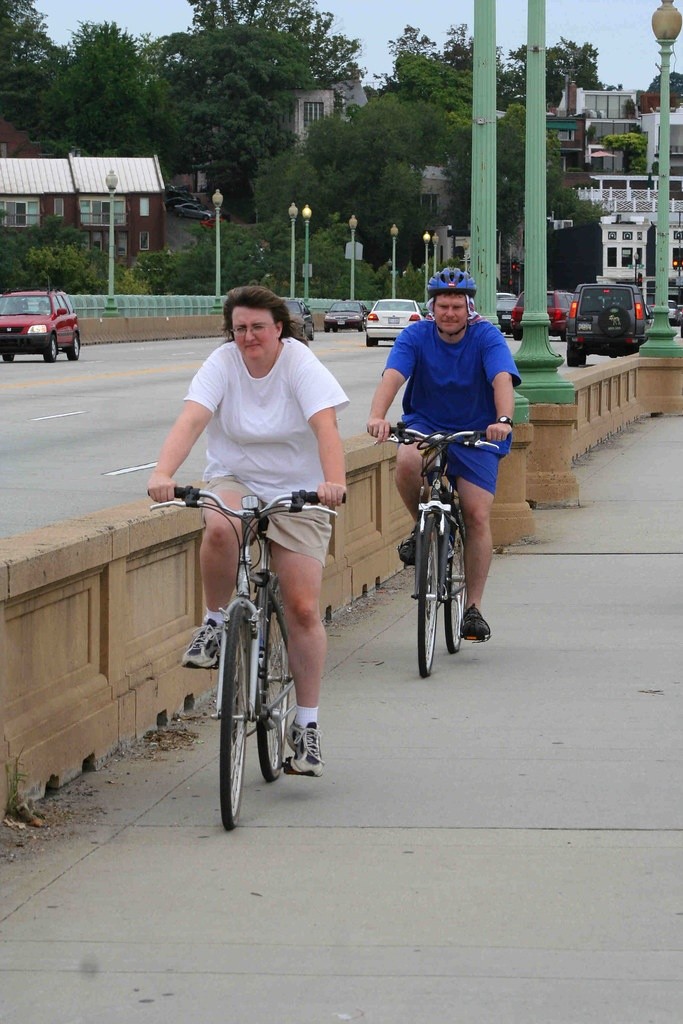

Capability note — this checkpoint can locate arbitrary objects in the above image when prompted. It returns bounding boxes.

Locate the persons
[146,286,350,778]
[39,301,47,310]
[366,269,523,640]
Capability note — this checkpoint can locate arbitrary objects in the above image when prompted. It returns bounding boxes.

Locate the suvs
[495,284,683,368]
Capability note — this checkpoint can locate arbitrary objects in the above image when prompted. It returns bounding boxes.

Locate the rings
[498,438,501,439]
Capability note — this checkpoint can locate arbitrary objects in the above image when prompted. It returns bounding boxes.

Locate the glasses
[230,323,274,336]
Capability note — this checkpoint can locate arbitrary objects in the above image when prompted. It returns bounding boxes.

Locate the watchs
[496,416,513,427]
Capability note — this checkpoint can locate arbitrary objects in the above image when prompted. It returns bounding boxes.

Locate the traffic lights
[673,248,683,267]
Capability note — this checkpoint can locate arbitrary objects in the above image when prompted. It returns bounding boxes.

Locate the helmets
[427,268,477,297]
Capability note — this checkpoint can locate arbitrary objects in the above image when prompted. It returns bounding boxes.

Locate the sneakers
[399,525,416,565]
[460,603,490,638]
[181,615,222,668]
[287,714,325,776]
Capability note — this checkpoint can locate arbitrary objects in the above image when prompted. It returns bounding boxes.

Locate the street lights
[301,205,313,313]
[634,252,638,285]
[639,0,683,359]
[288,203,298,299]
[464,241,469,273]
[423,232,430,302]
[350,215,357,301]
[390,224,398,299]
[211,189,225,314]
[101,168,122,317]
[432,233,439,277]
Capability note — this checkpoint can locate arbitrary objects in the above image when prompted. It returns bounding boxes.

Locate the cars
[366,299,425,347]
[0,289,81,362]
[282,298,314,341]
[323,302,371,332]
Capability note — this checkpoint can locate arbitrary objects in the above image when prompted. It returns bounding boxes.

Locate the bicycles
[367,422,499,678]
[147,485,347,831]
